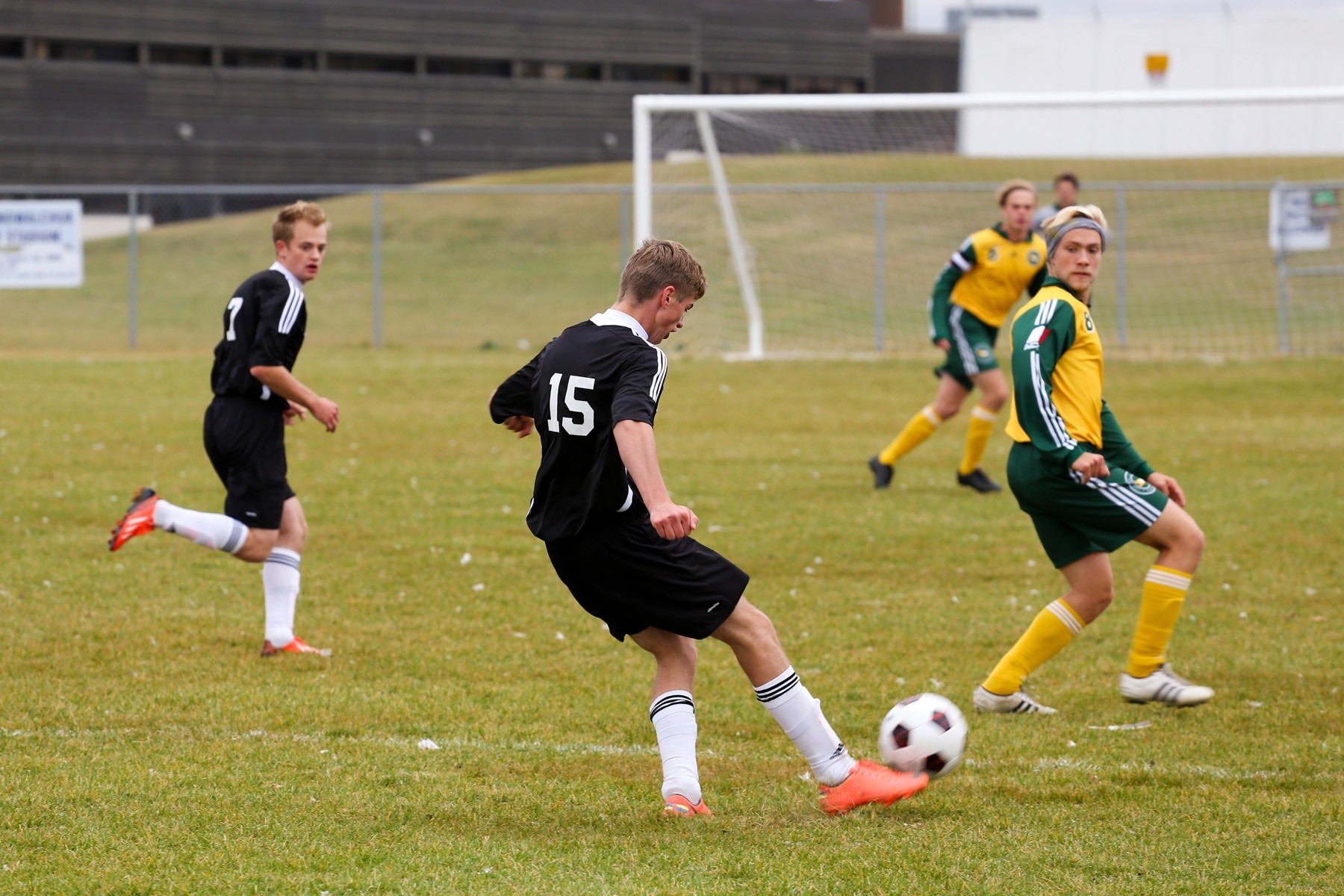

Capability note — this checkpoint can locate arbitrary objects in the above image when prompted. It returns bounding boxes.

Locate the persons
[865,179,1049,494]
[488,235,929,818]
[106,201,344,661]
[1033,171,1080,229]
[970,206,1215,719]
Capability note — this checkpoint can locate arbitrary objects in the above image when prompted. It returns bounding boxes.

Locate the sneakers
[260,635,334,659]
[972,685,1058,715]
[818,760,930,816]
[108,486,161,552]
[1120,662,1216,708]
[867,454,895,489]
[662,793,714,818]
[956,466,1004,492]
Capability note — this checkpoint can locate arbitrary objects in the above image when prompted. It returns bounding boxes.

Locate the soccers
[879,694,967,780]
